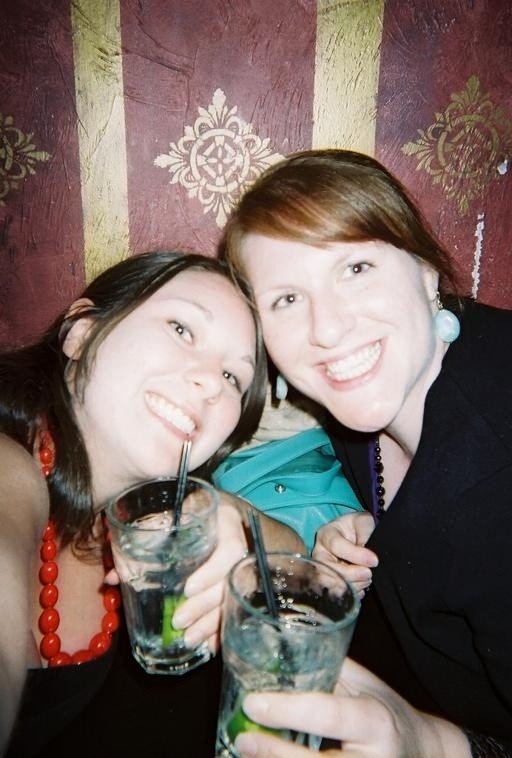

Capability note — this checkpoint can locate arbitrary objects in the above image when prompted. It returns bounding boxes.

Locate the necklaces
[372,432,386,520]
[32,427,121,671]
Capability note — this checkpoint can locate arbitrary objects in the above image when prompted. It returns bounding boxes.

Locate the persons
[1,238,310,758]
[214,147,512,756]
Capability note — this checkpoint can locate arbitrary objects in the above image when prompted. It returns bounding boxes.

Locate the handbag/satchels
[208,427,367,552]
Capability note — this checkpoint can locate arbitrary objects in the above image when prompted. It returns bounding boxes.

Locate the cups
[104,474,223,676]
[213,550,361,756]
